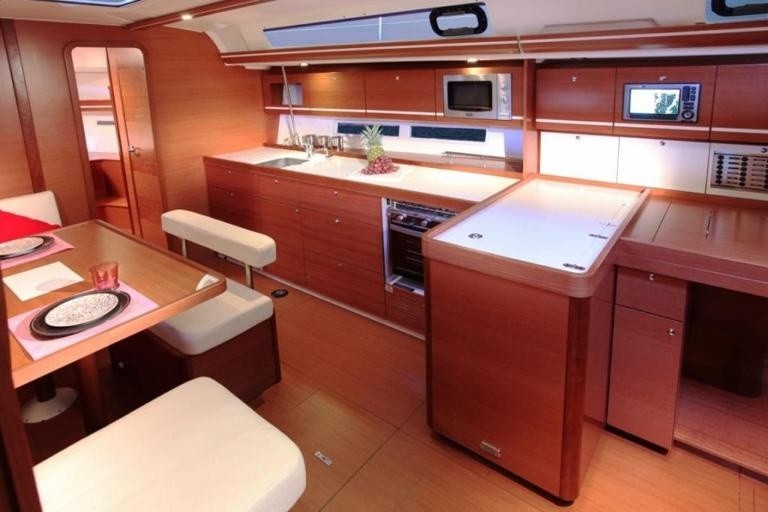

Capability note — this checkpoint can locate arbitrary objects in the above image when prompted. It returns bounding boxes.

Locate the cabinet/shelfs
[302,173,384,324]
[261,62,525,132]
[605,264,688,452]
[203,153,306,294]
[533,62,768,144]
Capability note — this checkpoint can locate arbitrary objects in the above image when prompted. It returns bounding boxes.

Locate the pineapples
[359,122,385,162]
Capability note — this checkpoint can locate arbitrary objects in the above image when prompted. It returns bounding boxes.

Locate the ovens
[380,196,462,296]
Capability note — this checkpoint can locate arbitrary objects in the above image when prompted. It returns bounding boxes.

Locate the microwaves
[442,74,513,123]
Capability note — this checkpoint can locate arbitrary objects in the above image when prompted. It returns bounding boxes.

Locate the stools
[32,376,306,512]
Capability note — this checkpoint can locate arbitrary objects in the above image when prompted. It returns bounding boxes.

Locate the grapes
[361,154,399,176]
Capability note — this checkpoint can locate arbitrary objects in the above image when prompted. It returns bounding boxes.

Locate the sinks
[256,157,308,169]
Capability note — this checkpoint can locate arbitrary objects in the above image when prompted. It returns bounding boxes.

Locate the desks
[0,219,226,429]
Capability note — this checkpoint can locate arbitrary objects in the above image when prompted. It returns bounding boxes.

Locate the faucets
[301,142,314,157]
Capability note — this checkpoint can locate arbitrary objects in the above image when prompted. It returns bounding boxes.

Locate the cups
[304,134,344,152]
[87,260,120,292]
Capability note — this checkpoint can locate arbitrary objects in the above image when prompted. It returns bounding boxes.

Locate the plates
[43,292,117,328]
[0,236,44,257]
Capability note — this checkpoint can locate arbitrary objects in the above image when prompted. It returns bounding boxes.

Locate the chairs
[0,189,62,245]
[108,208,281,403]
